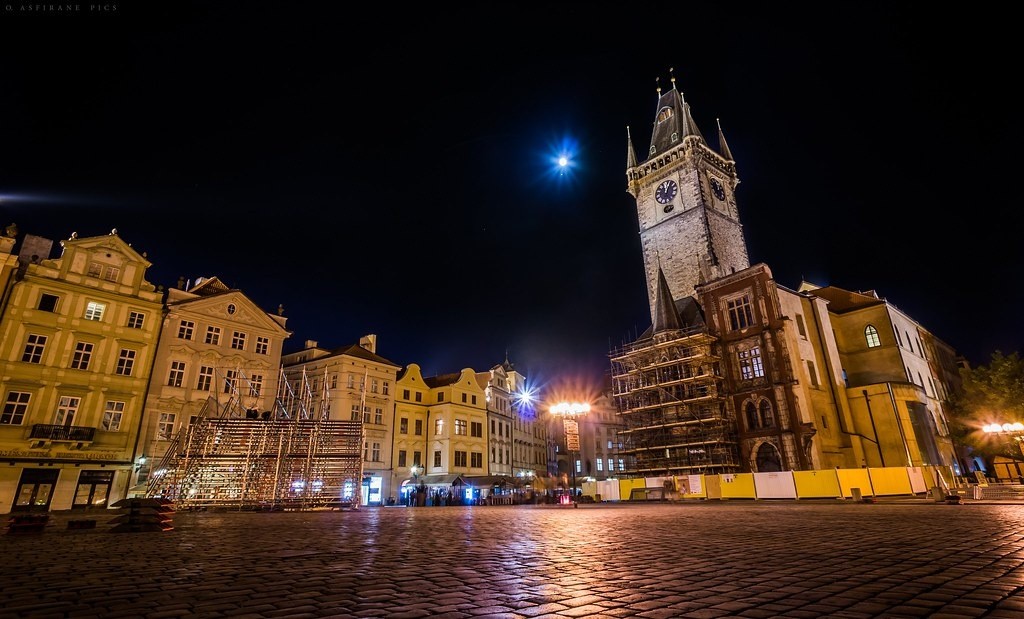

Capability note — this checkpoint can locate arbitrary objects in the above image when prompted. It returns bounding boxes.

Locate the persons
[410,489,582,506]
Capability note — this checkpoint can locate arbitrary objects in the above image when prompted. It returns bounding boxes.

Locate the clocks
[655,180,678,204]
[710,178,725,202]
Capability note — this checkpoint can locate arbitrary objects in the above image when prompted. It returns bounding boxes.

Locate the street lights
[547,400,592,508]
[510,393,530,478]
[982,422,1024,485]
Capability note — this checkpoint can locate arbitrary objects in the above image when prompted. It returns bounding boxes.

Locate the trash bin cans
[931,486,944,501]
[595,494,600,502]
[850,488,862,501]
